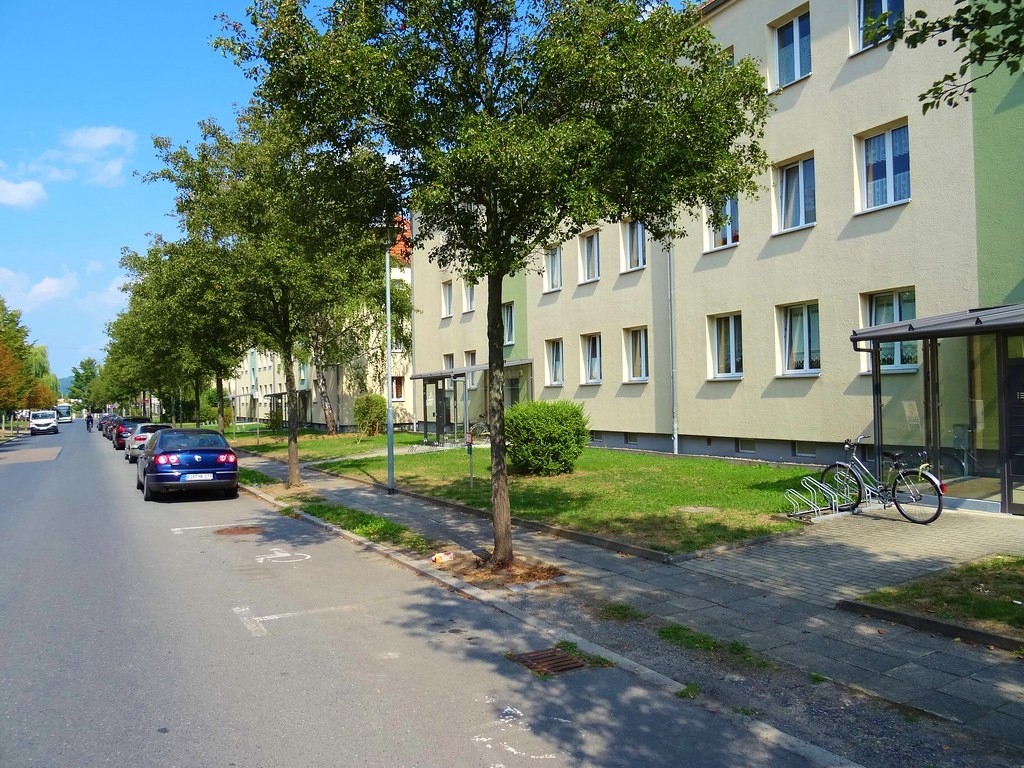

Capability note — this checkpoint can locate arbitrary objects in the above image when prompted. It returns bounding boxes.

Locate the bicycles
[941,430,1000,480]
[85,420,93,432]
[820,435,946,524]
[469,414,490,445]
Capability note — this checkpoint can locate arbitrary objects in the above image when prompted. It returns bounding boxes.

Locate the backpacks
[87,414,91,417]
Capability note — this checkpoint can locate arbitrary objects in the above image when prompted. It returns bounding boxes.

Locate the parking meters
[466,432,473,490]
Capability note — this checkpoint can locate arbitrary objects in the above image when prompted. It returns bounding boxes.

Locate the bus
[55,404,73,423]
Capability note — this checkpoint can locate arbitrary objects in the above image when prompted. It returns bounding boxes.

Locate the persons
[85,411,93,430]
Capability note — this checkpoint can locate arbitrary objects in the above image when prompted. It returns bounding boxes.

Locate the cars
[137,429,239,501]
[97,413,152,450]
[125,423,175,463]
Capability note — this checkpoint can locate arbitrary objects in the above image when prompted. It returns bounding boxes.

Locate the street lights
[376,224,407,495]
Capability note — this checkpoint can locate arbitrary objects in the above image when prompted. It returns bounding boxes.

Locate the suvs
[29,411,58,436]
